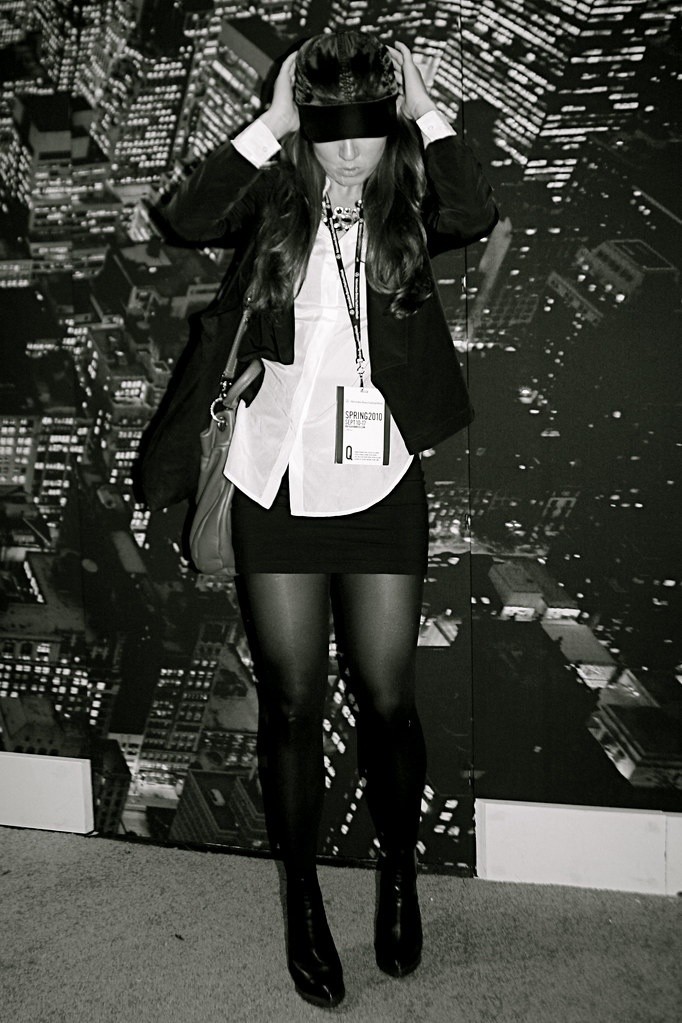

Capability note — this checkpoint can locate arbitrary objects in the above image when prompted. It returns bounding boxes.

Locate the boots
[277,859,346,1007]
[374,847,424,977]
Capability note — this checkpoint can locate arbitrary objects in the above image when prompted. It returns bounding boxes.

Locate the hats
[294,28,399,142]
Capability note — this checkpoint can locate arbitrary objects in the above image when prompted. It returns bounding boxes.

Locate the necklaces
[321,202,363,230]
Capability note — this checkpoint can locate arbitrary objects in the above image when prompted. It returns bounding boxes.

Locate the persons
[159,31,499,1006]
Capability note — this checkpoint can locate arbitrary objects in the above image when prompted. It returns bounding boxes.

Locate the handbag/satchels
[128,193,269,511]
[188,401,237,576]
